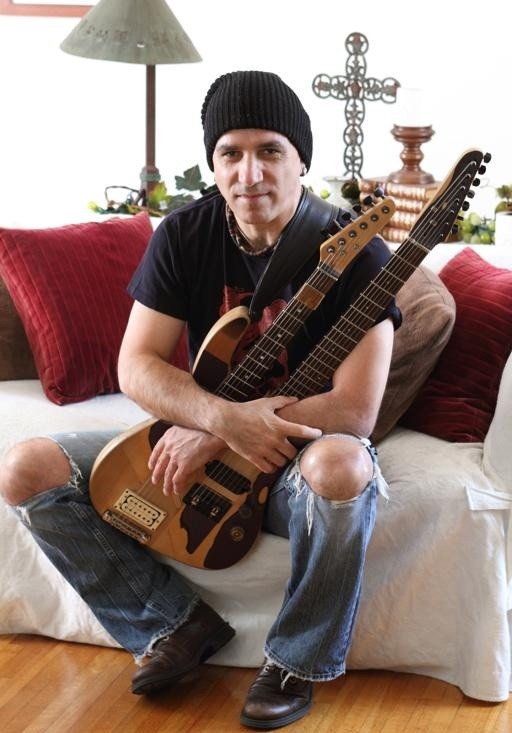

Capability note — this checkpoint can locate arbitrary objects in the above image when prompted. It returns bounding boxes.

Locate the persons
[1,70,404,730]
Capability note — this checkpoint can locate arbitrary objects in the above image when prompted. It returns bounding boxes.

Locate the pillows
[0,210,191,406]
[368,246,512,445]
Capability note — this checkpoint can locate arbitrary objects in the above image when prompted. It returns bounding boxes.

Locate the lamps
[59,0,204,209]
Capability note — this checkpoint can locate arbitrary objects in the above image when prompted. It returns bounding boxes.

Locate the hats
[201,71,312,169]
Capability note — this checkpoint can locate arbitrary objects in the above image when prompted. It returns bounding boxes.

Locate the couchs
[0,242,512,704]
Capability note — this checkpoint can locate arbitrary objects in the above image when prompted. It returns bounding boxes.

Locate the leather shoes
[130,598,237,694]
[240,658,311,729]
